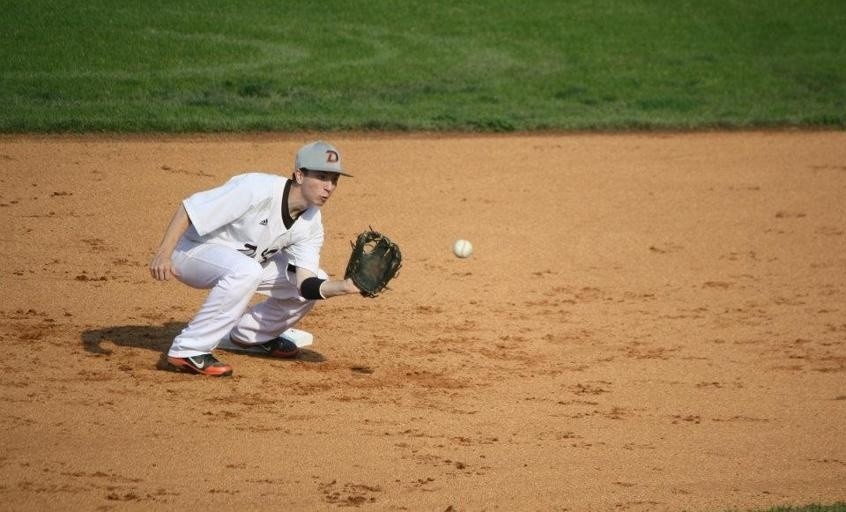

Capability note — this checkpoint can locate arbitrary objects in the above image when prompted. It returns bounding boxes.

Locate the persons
[149,138,363,379]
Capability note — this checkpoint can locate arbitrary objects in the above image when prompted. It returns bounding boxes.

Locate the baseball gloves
[344,230,401,297]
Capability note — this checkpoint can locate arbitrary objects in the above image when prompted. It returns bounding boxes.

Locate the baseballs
[454,239,472,257]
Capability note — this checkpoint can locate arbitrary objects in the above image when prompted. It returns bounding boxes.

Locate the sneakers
[167,349,233,378]
[227,326,298,359]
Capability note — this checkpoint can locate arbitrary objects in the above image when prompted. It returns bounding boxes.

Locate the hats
[292,139,355,180]
